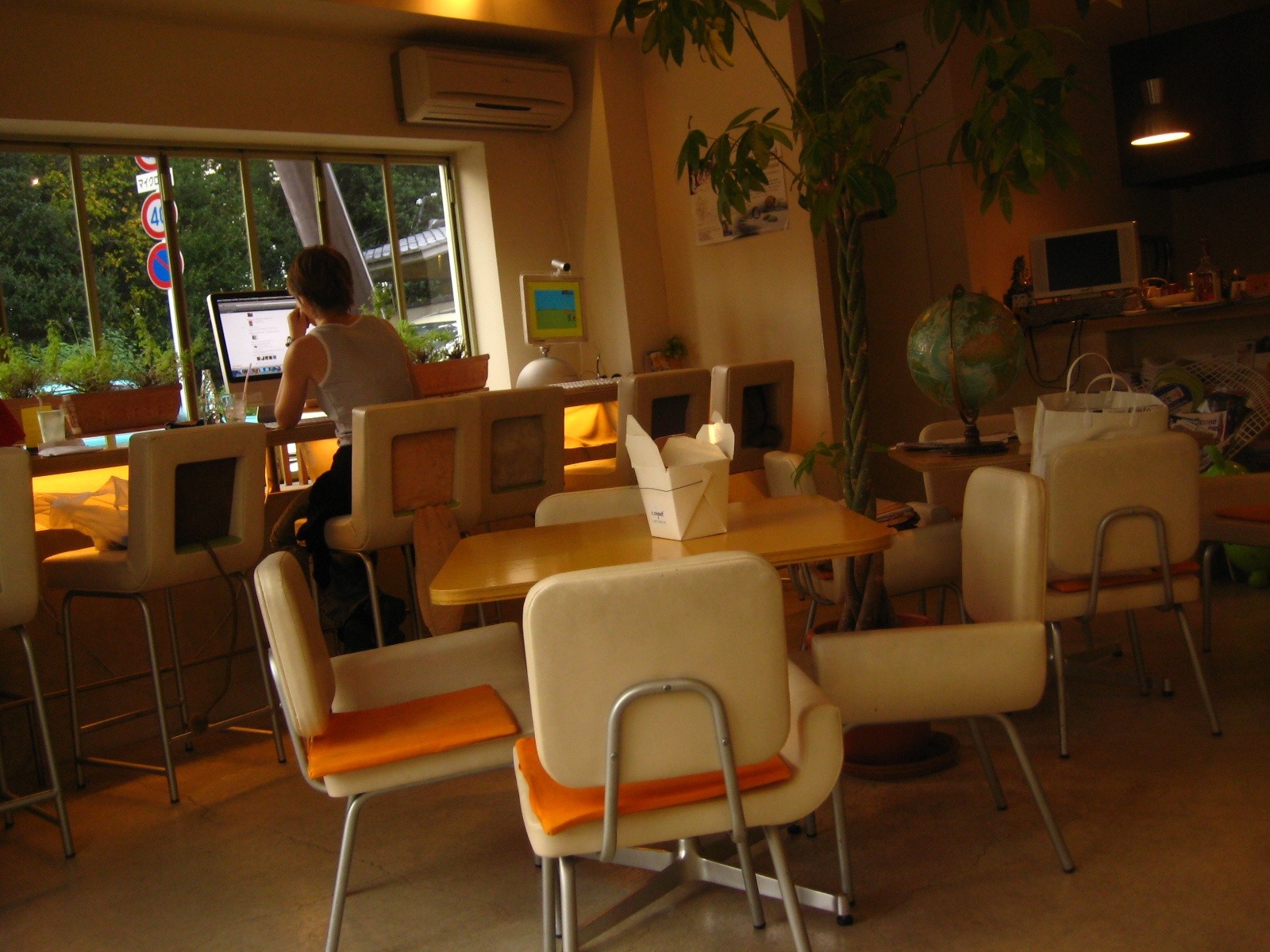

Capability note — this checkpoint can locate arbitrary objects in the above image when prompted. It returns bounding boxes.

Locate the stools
[309,394,497,656]
[712,352,799,474]
[476,380,571,534]
[41,418,282,805]
[0,446,79,866]
[566,362,719,490]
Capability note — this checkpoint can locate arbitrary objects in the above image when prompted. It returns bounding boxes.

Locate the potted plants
[0,298,184,441]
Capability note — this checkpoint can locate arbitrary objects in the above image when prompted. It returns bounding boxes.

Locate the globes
[906,283,1027,456]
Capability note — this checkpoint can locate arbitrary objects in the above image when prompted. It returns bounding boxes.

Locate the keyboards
[548,375,621,393]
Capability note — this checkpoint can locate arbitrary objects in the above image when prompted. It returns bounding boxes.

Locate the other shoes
[333,594,407,656]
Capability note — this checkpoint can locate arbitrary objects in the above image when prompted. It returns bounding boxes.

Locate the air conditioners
[389,44,576,133]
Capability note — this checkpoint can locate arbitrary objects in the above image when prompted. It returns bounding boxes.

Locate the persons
[271,244,412,655]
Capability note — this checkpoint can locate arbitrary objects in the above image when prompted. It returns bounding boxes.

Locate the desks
[884,433,1123,698]
[423,496,898,952]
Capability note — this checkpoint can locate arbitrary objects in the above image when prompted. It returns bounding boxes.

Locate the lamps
[1125,0,1198,150]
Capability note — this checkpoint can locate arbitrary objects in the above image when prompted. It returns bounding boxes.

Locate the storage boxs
[609,400,738,540]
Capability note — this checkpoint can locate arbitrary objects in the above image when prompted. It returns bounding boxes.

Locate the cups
[222,392,247,423]
[36,409,65,443]
[1012,405,1036,444]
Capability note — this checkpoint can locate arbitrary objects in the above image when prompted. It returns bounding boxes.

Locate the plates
[1147,292,1195,309]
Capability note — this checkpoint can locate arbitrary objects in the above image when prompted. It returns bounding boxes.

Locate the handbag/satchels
[1028,353,1169,583]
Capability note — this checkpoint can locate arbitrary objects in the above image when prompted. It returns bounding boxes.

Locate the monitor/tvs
[520,270,591,344]
[206,290,317,384]
[1027,220,1143,298]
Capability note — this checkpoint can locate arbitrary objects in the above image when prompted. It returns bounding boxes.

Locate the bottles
[200,368,221,425]
[1193,238,1221,301]
[59,395,83,438]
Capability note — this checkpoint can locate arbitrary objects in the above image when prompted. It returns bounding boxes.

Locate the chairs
[239,542,558,952]
[506,542,845,952]
[1029,431,1224,761]
[756,442,968,651]
[1195,463,1270,658]
[803,467,1084,901]
[532,482,646,529]
